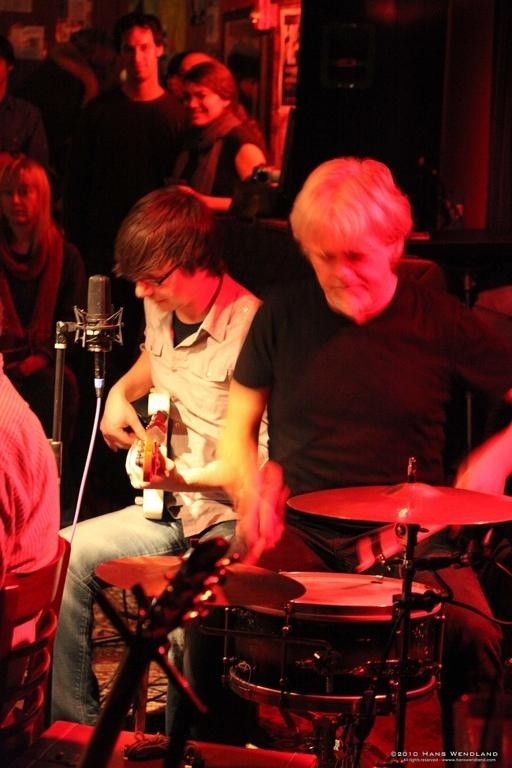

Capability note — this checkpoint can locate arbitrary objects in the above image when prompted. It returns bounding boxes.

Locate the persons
[1,353,60,725]
[48,184,270,741]
[0,13,284,522]
[154,153,512,740]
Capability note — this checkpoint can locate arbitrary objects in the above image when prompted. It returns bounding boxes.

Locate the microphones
[84,273,114,393]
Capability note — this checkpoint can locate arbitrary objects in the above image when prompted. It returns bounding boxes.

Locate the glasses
[149,261,183,289]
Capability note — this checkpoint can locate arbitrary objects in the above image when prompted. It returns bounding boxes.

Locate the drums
[227,572,442,700]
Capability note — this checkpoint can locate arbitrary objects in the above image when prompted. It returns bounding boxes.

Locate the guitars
[123,385,174,517]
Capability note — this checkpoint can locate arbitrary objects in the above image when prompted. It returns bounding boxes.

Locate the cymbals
[90,552,304,611]
[285,477,511,541]
